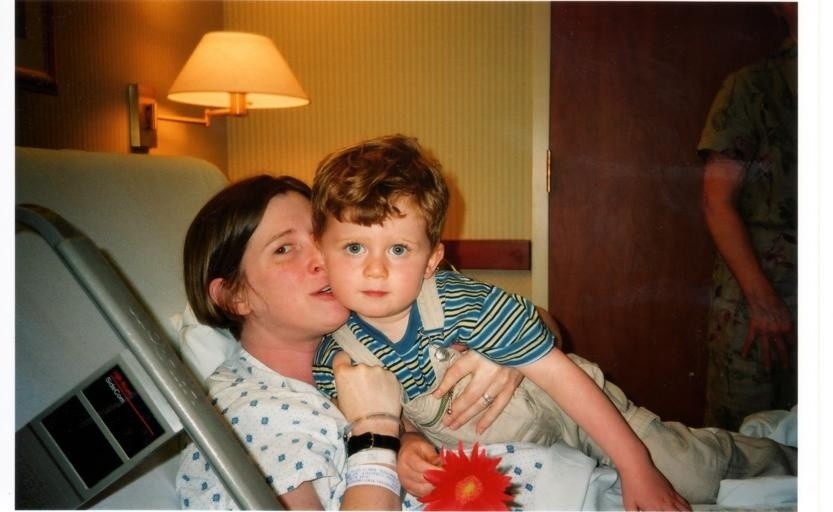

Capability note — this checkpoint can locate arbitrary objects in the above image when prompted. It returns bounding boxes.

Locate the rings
[482,393,493,404]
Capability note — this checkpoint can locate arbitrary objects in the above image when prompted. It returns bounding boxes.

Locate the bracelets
[342,413,404,435]
[341,465,401,496]
[345,450,398,468]
[345,430,403,456]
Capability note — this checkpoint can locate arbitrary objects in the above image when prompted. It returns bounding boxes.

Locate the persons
[696,3,798,431]
[309,131,797,511]
[172,173,619,511]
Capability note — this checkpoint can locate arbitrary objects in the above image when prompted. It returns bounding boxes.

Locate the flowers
[415,438,515,511]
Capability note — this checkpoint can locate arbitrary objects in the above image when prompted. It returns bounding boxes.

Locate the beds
[15,148,332,511]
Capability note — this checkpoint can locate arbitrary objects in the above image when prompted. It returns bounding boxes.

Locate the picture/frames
[15,0,63,100]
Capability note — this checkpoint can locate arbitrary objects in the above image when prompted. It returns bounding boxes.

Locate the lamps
[126,31,311,152]
